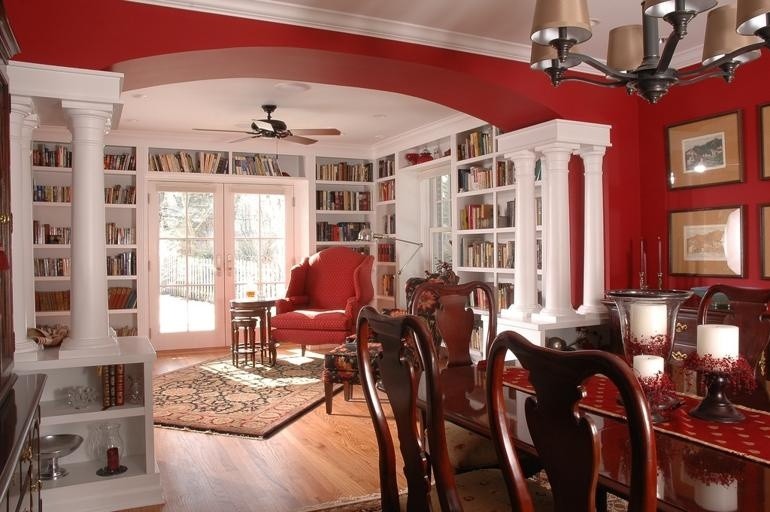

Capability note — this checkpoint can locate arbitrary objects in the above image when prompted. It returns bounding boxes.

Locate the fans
[192,102,342,158]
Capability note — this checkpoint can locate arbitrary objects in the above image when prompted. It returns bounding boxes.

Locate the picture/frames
[666,205,745,277]
[757,204,770,279]
[755,103,770,181]
[662,108,745,190]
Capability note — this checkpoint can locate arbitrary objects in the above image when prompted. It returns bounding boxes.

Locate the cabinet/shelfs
[148,141,310,189]
[596,296,769,380]
[7,335,168,512]
[310,146,415,331]
[27,123,150,343]
[0,0,48,512]
[454,122,541,357]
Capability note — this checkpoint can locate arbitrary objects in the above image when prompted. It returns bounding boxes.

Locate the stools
[231,318,257,367]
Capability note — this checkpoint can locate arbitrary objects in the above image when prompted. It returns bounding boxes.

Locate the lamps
[529,0,770,105]
[357,228,424,277]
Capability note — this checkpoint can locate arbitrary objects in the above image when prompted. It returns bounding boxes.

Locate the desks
[229,299,275,363]
[378,352,769,512]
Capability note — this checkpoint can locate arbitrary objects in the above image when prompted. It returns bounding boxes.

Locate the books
[471,319,484,356]
[463,237,514,269]
[109,365,117,405]
[497,282,513,312]
[106,222,136,245]
[537,239,542,269]
[458,133,492,161]
[108,287,137,309]
[105,153,136,170]
[149,150,229,174]
[537,198,542,226]
[233,152,282,176]
[33,256,71,276]
[497,160,516,185]
[316,190,371,210]
[499,198,515,227]
[317,221,370,242]
[354,247,371,254]
[535,159,541,181]
[104,184,136,204]
[383,214,395,234]
[378,243,395,262]
[107,250,136,275]
[381,181,395,200]
[379,159,395,178]
[469,282,494,310]
[383,273,395,297]
[33,220,72,244]
[102,365,111,407]
[32,143,73,168]
[33,184,71,202]
[460,204,499,229]
[35,290,70,312]
[458,165,493,192]
[317,162,373,182]
[117,364,125,406]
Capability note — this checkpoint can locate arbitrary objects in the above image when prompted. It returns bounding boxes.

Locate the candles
[696,323,740,371]
[628,300,669,348]
[693,465,739,512]
[621,455,665,502]
[630,351,664,393]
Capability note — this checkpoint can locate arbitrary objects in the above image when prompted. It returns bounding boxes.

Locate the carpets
[150,348,346,439]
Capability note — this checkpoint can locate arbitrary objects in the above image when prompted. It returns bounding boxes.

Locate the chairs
[411,281,497,372]
[697,285,770,368]
[356,306,466,512]
[486,332,657,511]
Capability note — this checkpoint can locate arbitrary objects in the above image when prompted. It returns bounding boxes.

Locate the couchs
[321,259,462,413]
[268,248,374,367]
